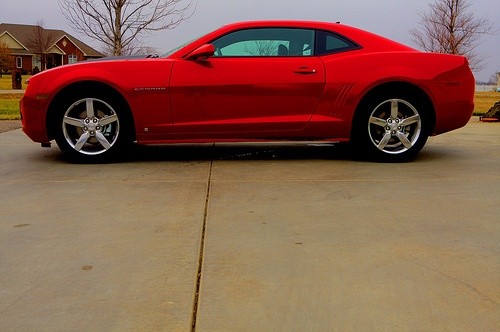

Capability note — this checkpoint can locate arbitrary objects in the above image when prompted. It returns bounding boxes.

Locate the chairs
[277,44,288,57]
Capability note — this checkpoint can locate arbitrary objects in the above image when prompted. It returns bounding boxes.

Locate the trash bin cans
[11,69,22,89]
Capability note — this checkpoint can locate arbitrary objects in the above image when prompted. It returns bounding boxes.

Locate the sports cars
[18,19,477,164]
